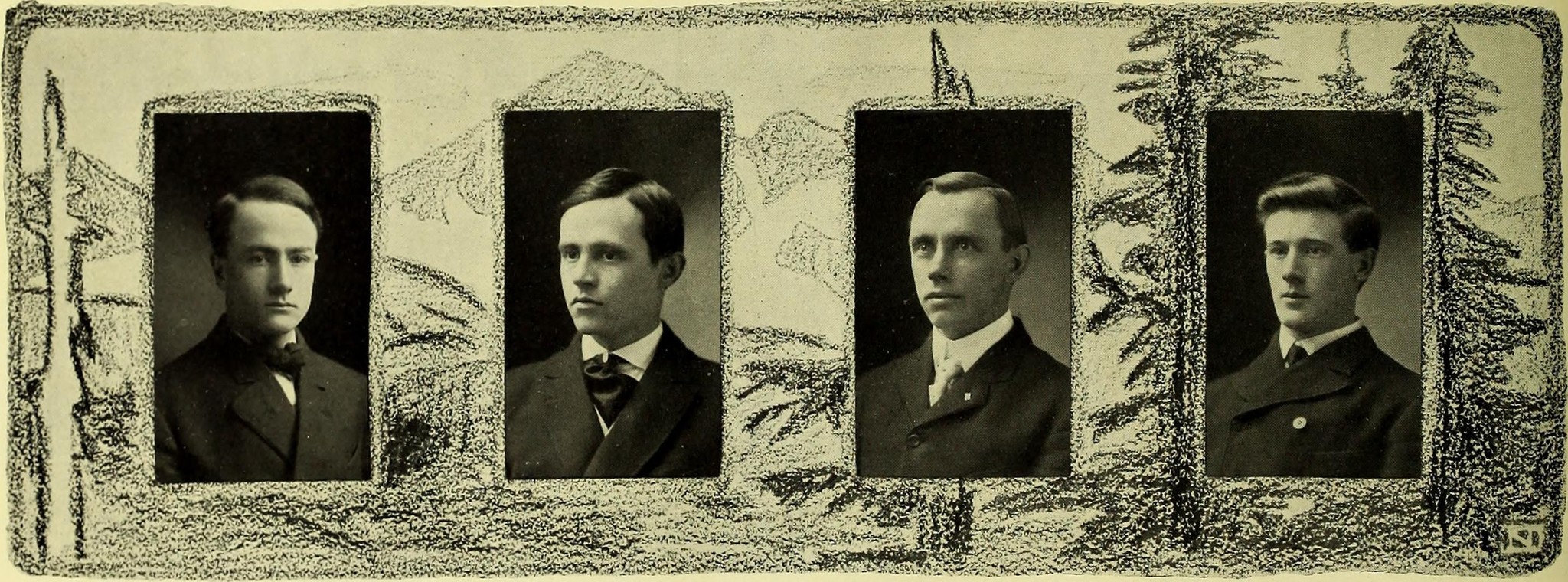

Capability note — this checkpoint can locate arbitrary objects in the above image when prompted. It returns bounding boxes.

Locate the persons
[857,166,1070,475]
[505,169,722,477]
[154,175,370,483]
[1204,171,1422,476]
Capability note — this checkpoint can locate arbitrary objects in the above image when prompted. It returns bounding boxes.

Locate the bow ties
[262,343,305,376]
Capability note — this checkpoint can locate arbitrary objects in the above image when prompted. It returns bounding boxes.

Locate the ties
[1287,345,1307,369]
[583,354,642,426]
[932,359,963,405]
